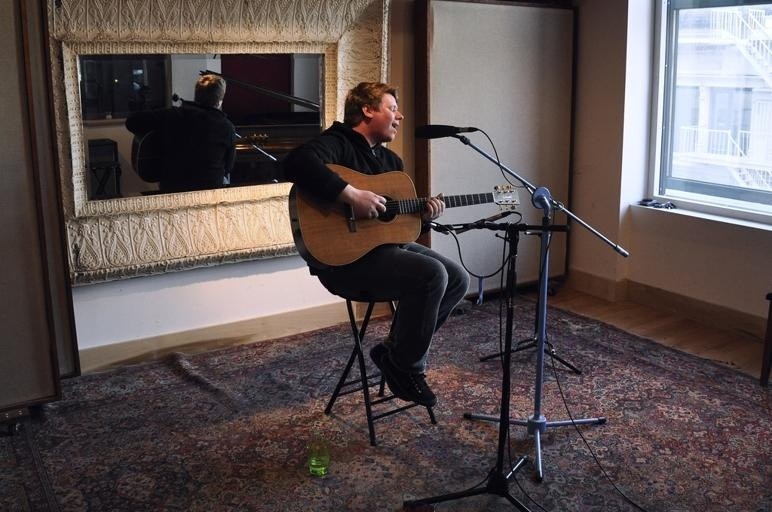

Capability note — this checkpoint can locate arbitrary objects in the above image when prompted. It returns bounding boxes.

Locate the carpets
[8,293,772,512]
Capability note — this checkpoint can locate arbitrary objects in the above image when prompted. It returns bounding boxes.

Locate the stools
[324,289,437,446]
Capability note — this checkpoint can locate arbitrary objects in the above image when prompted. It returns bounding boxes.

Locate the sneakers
[370,344,437,406]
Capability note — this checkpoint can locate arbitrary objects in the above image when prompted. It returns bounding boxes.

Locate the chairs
[88,138,120,199]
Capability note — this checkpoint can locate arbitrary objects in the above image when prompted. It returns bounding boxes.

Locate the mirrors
[77,53,324,202]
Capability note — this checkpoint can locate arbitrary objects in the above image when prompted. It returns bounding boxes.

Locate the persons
[124,72,237,191]
[279,80,471,409]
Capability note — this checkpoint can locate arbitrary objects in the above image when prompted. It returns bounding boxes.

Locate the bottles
[307,427,331,477]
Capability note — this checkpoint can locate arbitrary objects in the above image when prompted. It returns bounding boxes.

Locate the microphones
[456,210,510,233]
[416,124,476,140]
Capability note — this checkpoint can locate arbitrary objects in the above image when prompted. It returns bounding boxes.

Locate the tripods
[481,273,582,375]
[464,266,606,483]
[404,294,529,512]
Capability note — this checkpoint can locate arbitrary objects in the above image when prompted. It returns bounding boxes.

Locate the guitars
[131,127,269,183]
[288,164,520,267]
